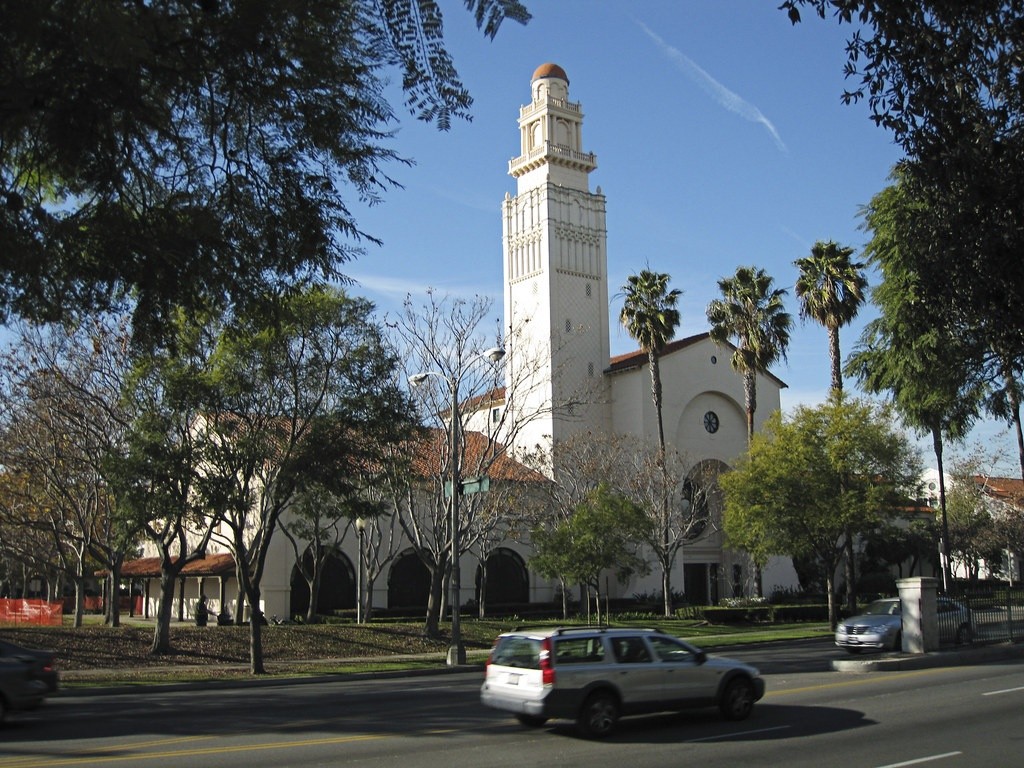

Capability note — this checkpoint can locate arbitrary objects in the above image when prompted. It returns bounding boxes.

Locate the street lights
[355,517,365,625]
[407,345,508,667]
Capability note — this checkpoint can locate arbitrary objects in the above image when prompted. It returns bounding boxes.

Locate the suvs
[481,624,761,739]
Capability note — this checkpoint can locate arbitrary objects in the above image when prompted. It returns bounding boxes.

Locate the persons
[194,595,215,626]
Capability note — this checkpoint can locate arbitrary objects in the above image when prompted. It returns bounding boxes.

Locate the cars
[0,640,59,720]
[833,595,978,651]
